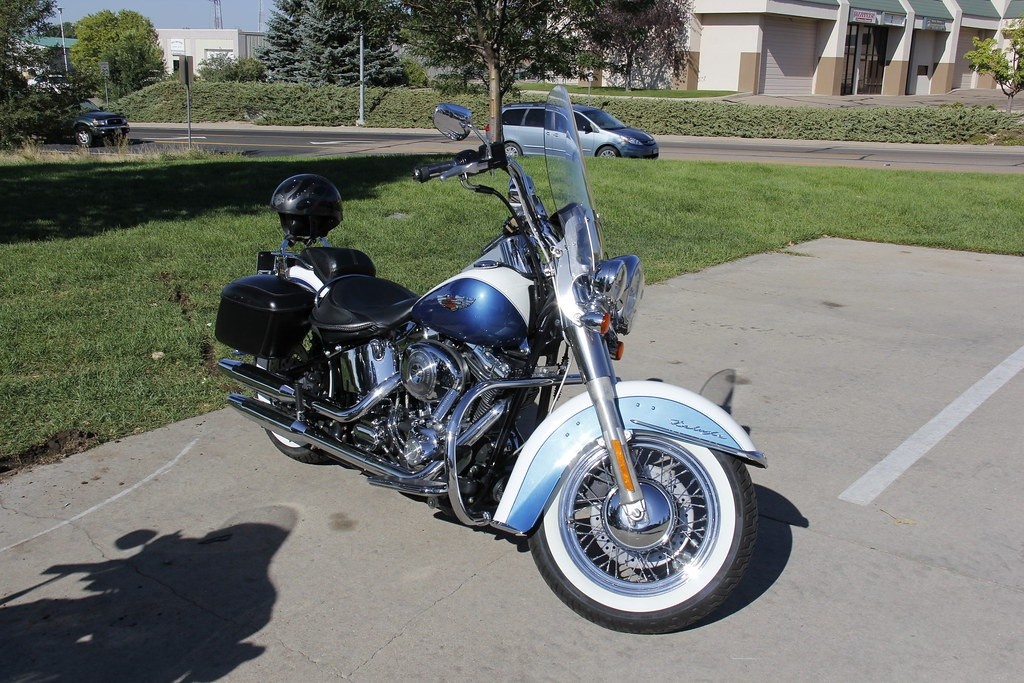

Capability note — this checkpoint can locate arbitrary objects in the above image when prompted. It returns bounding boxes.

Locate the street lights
[55,6,68,74]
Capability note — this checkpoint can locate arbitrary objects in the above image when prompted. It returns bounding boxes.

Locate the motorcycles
[213,84,768,635]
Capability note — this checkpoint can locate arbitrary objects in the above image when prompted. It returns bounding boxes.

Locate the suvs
[46,98,131,148]
[485,101,660,159]
